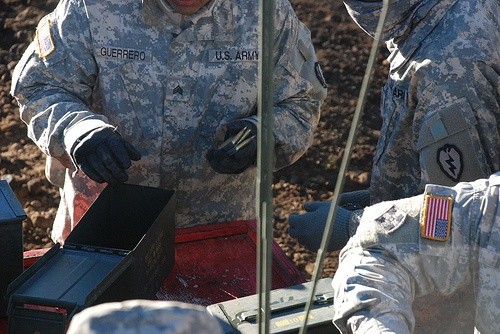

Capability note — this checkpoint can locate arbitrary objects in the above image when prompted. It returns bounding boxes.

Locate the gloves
[288,201,350,252]
[70,122,141,190]
[329,190,369,211]
[207,121,257,174]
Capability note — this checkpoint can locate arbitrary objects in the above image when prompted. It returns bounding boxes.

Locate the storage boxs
[0,180,27,318]
[7,183,176,334]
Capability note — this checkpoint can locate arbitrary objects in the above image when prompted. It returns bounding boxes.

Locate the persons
[11,0,328,246]
[288,0,500,250]
[331,172,500,334]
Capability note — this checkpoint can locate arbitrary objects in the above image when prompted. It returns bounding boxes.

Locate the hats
[66,299,225,334]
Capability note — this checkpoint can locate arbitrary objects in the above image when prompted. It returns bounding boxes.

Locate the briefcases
[206,277,336,334]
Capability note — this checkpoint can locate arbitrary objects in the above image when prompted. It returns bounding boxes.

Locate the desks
[0,218,309,334]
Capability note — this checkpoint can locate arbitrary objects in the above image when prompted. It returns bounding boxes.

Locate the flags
[421,195,452,241]
